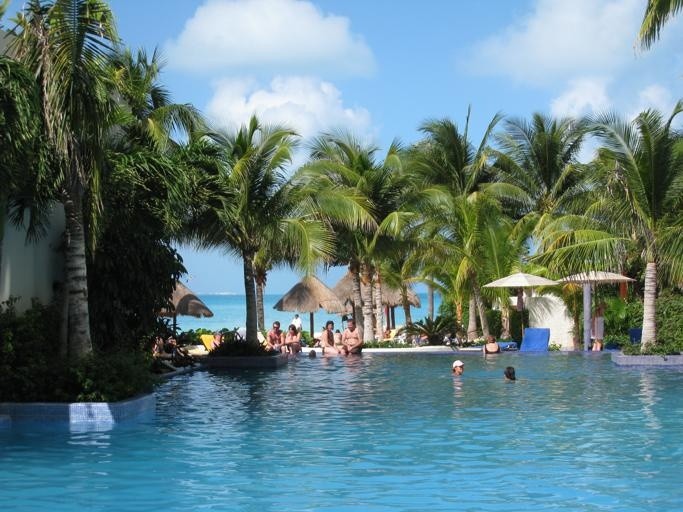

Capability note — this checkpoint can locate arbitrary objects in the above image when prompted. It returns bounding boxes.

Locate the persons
[482,335,502,354]
[210,332,225,350]
[307,349,316,359]
[309,338,320,347]
[320,320,340,357]
[502,365,515,381]
[334,329,341,345]
[451,359,465,376]
[590,301,609,352]
[163,342,195,367]
[265,320,286,358]
[284,324,302,358]
[290,313,302,333]
[340,317,363,357]
[381,329,464,346]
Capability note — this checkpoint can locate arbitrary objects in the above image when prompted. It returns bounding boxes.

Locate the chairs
[201,333,217,353]
[170,345,195,371]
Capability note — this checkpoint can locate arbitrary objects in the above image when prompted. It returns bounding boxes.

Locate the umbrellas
[480,271,560,336]
[554,268,637,351]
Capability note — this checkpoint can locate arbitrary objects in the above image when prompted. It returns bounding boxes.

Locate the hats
[453,360,464,368]
